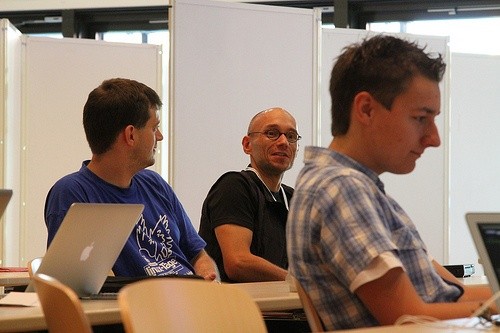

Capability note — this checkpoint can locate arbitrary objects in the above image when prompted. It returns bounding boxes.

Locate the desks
[456,275,490,287]
[323,314,500,333]
[0,280,303,333]
[0,271,32,288]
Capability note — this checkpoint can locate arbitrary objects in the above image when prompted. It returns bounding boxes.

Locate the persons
[285,33,500,333]
[44,78,221,284]
[198,107,302,283]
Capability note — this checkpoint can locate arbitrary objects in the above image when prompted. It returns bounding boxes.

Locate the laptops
[466,212,500,314]
[4,203,144,299]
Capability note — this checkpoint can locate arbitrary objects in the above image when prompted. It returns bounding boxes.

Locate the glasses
[247,128,308,146]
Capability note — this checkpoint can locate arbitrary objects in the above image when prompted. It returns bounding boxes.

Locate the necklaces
[245,167,289,212]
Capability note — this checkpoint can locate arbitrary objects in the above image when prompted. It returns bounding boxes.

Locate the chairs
[289,273,323,333]
[32,273,92,333]
[117,277,269,333]
